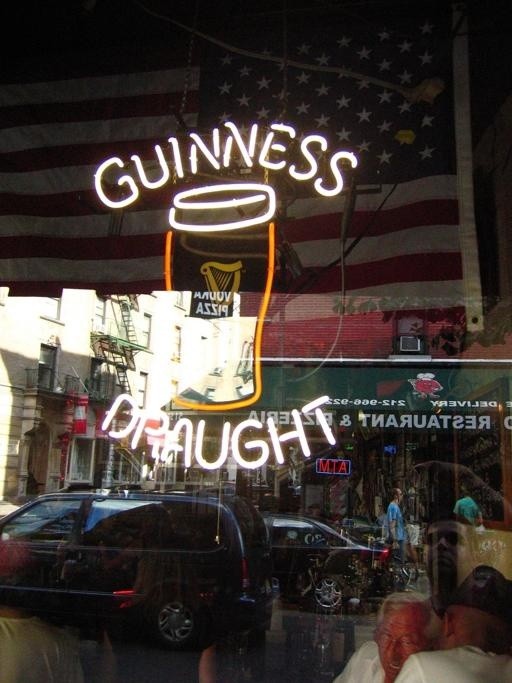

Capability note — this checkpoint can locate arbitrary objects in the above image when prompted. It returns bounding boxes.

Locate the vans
[0,478,281,646]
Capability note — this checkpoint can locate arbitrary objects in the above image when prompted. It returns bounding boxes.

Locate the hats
[448,563,512,627]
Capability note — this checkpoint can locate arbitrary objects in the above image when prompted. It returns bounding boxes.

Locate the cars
[255,505,393,612]
[155,462,299,498]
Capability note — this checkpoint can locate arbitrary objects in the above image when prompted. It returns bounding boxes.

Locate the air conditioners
[398,334,423,353]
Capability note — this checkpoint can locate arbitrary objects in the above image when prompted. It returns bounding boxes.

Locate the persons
[386,487,414,592]
[1,536,87,682]
[453,487,483,526]
[423,511,477,603]
[332,588,434,682]
[394,564,512,683]
[55,500,219,683]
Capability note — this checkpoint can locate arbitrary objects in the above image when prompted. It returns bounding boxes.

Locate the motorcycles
[273,551,345,608]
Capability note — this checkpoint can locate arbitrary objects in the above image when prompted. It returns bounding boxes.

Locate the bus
[411,374,512,620]
